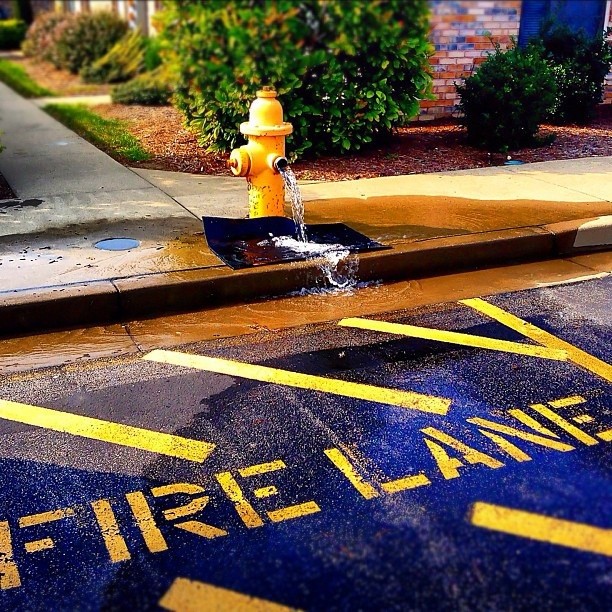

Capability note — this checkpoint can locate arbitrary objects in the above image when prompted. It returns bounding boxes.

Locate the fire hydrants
[227,86,293,219]
